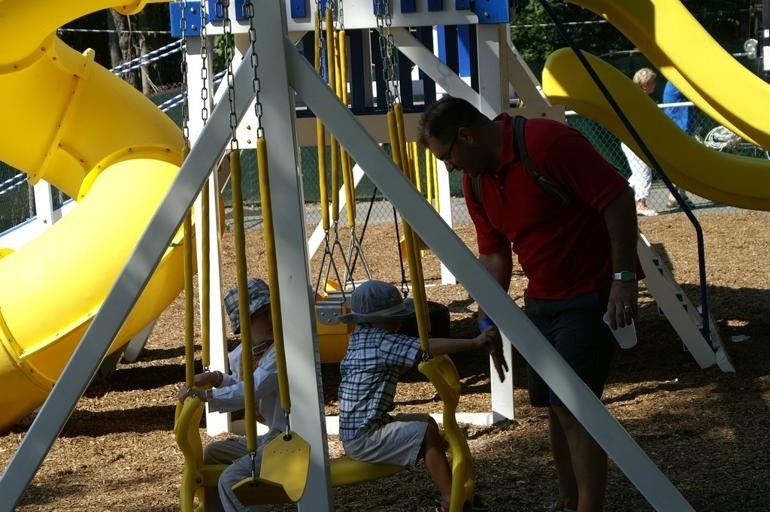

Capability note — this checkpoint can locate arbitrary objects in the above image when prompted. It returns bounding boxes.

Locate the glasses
[439,126,460,163]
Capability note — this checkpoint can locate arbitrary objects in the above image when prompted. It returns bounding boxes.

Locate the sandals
[635,198,689,217]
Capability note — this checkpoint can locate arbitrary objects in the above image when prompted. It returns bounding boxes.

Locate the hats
[223,278,273,335]
[336,278,421,326]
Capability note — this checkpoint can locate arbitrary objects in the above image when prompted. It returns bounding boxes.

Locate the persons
[336,279,502,511]
[176,277,290,512]
[620,68,659,217]
[416,96,640,512]
[661,80,696,212]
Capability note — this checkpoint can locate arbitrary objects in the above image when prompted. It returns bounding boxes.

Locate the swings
[313,2,479,511]
[173,1,457,512]
[308,2,444,379]
[220,2,312,502]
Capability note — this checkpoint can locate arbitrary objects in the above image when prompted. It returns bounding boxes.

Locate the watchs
[611,270,637,282]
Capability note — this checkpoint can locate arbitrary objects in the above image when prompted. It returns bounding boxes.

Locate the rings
[623,305,631,312]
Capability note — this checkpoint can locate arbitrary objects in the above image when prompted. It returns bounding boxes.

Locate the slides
[543,0,770,210]
[0,0,226,433]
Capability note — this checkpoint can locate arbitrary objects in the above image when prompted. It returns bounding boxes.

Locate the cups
[603,310,638,350]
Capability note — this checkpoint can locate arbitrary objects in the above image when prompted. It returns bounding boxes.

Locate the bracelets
[478,319,493,331]
